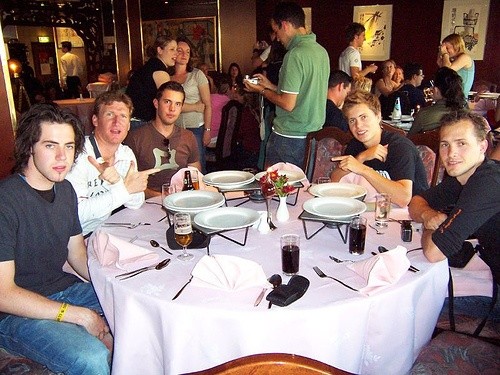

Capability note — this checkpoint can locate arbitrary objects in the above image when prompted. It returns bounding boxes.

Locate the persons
[330,89,430,207]
[125,33,205,130]
[408,66,470,133]
[410,109,500,321]
[197,63,217,94]
[388,65,426,116]
[210,79,231,144]
[60,40,83,100]
[255,2,406,171]
[228,62,243,95]
[123,81,202,198]
[63,89,160,239]
[436,33,474,99]
[169,37,211,174]
[0,101,113,375]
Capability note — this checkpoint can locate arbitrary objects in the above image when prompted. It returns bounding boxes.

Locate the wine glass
[173,213,194,261]
[423,87,433,102]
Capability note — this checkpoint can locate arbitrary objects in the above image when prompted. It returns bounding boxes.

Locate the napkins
[267,161,310,189]
[339,172,385,202]
[93,228,158,271]
[473,98,496,111]
[347,245,411,287]
[190,253,272,300]
[170,167,217,193]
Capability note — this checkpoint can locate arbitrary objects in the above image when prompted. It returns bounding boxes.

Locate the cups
[348,216,367,256]
[190,170,199,190]
[243,168,256,195]
[162,183,176,206]
[280,234,300,277]
[317,177,330,184]
[375,193,391,228]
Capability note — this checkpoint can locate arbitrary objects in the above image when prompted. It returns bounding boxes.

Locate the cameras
[401,222,412,242]
[243,75,259,86]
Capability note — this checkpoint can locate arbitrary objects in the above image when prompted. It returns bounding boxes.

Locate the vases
[275,196,289,221]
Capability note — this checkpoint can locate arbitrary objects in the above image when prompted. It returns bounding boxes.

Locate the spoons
[378,246,420,272]
[329,255,343,264]
[120,259,171,280]
[369,224,384,235]
[267,273,282,309]
[150,240,173,255]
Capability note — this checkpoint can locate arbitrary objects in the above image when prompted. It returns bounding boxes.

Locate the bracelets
[56,302,69,322]
[205,128,211,131]
[253,48,259,53]
[442,53,449,57]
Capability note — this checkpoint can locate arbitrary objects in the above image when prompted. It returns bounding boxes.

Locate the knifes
[371,252,417,272]
[254,277,271,307]
[104,222,152,225]
[115,262,161,277]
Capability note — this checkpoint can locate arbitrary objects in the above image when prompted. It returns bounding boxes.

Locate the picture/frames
[353,5,393,62]
[441,0,490,61]
[142,16,217,71]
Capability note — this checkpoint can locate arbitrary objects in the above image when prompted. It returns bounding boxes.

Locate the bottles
[400,220,413,242]
[390,97,401,125]
[181,171,196,191]
[415,105,421,114]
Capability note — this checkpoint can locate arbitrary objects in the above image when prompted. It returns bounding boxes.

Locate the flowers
[261,169,295,196]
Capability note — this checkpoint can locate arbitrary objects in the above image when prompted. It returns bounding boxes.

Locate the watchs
[261,90,266,94]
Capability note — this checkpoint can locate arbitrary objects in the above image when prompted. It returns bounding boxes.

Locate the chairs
[302,125,350,185]
[412,316,500,375]
[207,99,245,171]
[407,130,440,188]
[473,80,498,94]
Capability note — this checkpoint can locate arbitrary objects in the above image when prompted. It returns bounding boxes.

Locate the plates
[202,170,306,189]
[163,190,260,230]
[308,181,368,198]
[302,197,367,219]
[389,115,413,121]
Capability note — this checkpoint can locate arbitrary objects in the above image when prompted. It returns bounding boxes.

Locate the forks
[312,266,358,292]
[101,223,142,229]
[128,235,139,243]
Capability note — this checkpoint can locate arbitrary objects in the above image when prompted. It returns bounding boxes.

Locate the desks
[86,80,115,97]
[54,99,97,136]
[86,181,450,375]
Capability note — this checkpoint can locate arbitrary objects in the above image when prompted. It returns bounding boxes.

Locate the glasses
[162,138,171,159]
